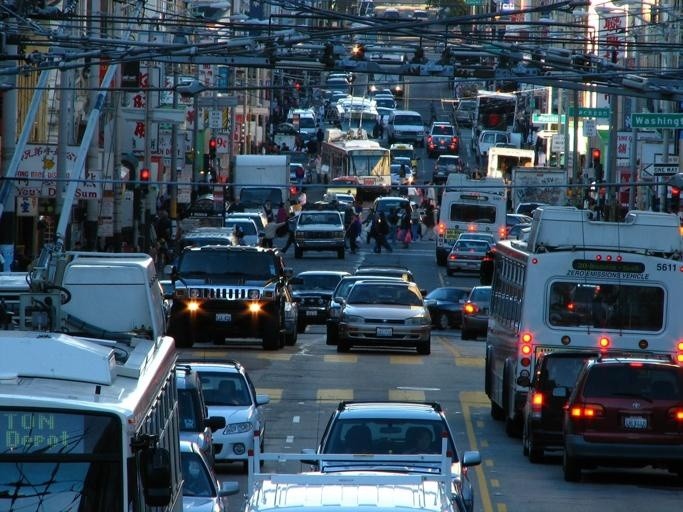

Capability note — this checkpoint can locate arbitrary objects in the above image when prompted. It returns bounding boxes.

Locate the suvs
[175,366,226,473]
[300,113,316,137]
[561,357,682,481]
[175,226,239,247]
[227,210,268,242]
[223,218,265,248]
[373,98,397,116]
[434,154,465,177]
[284,164,306,189]
[169,245,304,350]
[280,151,313,182]
[287,270,351,333]
[373,196,415,239]
[411,10,430,28]
[383,9,400,26]
[453,99,477,124]
[521,348,657,464]
[178,442,239,512]
[457,232,496,249]
[354,266,427,296]
[389,144,420,174]
[294,209,347,259]
[298,401,483,511]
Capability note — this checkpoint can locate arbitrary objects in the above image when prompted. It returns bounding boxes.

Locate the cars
[423,287,473,329]
[426,125,459,157]
[158,280,176,334]
[329,94,349,111]
[185,363,271,472]
[390,164,414,184]
[392,156,416,176]
[506,213,535,241]
[446,240,492,276]
[652,172,683,213]
[459,286,491,339]
[333,193,356,209]
[334,279,433,354]
[327,78,351,93]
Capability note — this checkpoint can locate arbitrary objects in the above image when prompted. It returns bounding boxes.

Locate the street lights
[595,7,670,214]
[168,14,248,239]
[572,9,636,213]
[614,0,683,171]
[228,20,273,191]
[144,1,230,212]
[540,17,591,167]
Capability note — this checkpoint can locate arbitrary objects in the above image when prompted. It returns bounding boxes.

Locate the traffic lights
[670,187,680,211]
[293,83,303,90]
[592,148,602,180]
[138,167,153,194]
[209,138,216,161]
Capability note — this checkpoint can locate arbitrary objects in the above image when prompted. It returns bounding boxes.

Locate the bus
[484,207,683,438]
[471,90,519,150]
[366,52,407,99]
[1,252,184,512]
[447,45,498,93]
[435,173,508,265]
[321,275,406,344]
[321,140,391,199]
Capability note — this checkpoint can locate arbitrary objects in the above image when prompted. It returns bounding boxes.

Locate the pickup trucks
[475,130,519,162]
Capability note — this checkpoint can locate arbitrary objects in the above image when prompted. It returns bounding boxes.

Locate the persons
[578,172,635,223]
[245,122,343,185]
[347,155,439,253]
[278,207,308,257]
[260,218,287,247]
[472,166,482,181]
[101,195,176,270]
[428,99,439,126]
[270,84,342,126]
[11,245,32,272]
[179,178,216,222]
[227,195,245,213]
[258,189,340,225]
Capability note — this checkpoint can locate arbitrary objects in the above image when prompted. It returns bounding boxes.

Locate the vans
[387,110,425,146]
[343,101,370,110]
[516,203,550,221]
[325,176,360,205]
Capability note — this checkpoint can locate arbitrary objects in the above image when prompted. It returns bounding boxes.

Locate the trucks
[487,147,535,178]
[230,155,291,224]
[511,166,568,211]
[242,428,465,512]
